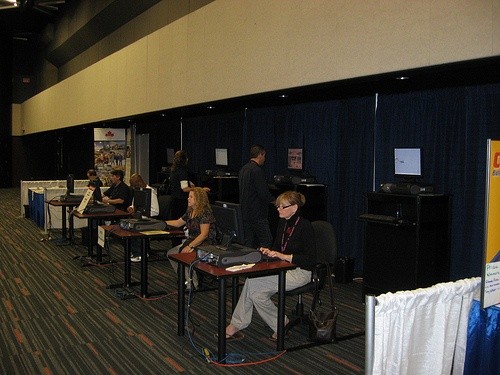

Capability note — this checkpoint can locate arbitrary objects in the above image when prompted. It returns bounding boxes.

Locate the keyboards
[165,224,176,231]
[259,251,278,263]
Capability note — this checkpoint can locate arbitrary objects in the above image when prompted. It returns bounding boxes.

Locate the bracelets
[187,244,194,249]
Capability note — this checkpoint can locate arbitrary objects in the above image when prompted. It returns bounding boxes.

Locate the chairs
[283,220,337,339]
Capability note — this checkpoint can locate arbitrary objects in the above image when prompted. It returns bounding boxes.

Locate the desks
[100,224,185,300]
[44,200,81,246]
[67,209,131,267]
[168,251,296,366]
[28,186,59,228]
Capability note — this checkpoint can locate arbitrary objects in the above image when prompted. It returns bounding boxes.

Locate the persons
[127,174,159,262]
[215,191,317,340]
[103,170,131,240]
[171,151,210,220]
[239,145,276,249]
[87,170,104,228]
[165,187,218,291]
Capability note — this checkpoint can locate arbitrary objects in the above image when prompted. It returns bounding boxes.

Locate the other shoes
[129,251,150,262]
[185,287,201,294]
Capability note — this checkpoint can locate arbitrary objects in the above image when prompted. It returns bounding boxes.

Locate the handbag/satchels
[304,299,339,342]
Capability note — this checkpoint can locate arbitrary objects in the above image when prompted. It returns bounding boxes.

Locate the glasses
[274,202,296,210]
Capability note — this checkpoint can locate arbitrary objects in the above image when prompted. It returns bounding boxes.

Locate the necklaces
[281,216,300,251]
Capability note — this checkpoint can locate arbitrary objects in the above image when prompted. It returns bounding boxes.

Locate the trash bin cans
[23,205,29,218]
[336,255,355,284]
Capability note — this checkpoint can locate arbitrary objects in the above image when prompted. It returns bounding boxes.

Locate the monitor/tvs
[211,201,245,251]
[94,186,104,204]
[65,174,75,196]
[391,146,423,178]
[133,186,152,222]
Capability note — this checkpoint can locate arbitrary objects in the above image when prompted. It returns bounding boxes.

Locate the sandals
[212,325,242,342]
[269,318,297,342]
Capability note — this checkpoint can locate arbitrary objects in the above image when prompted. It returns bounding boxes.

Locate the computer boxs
[60,195,84,202]
[86,204,116,214]
[194,244,263,268]
[382,182,435,194]
[120,219,167,231]
